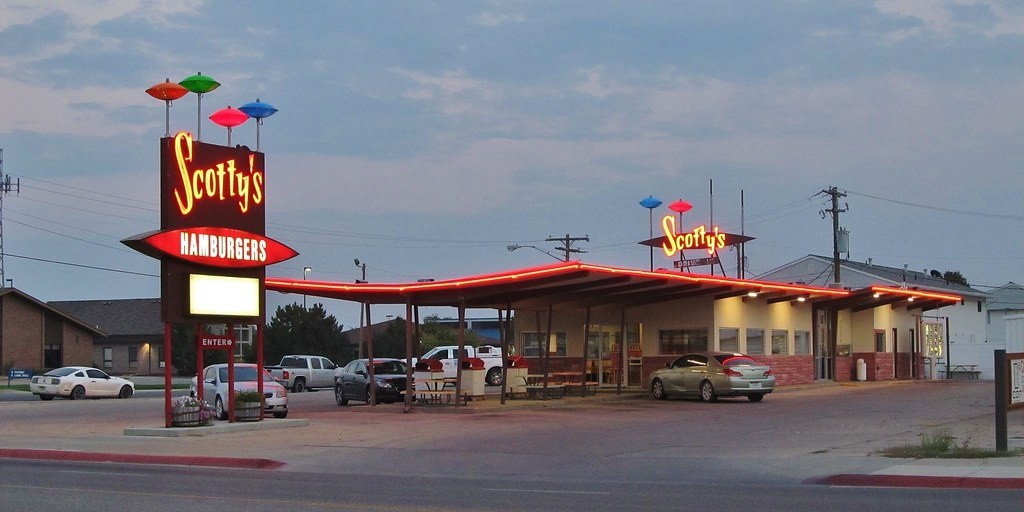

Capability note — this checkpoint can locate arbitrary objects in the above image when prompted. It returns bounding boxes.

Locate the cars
[648,350,776,403]
[29,365,135,400]
[189,363,289,418]
[333,357,416,406]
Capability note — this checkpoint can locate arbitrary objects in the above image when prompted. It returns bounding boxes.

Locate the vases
[172,407,201,427]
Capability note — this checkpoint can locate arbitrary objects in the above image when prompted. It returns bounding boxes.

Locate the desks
[548,371,594,397]
[413,379,457,405]
[943,364,980,380]
[512,374,559,400]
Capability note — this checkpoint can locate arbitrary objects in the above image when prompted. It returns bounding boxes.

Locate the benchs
[398,389,473,406]
[938,370,982,380]
[506,381,598,400]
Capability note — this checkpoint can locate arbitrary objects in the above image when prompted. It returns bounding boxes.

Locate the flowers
[171,395,217,426]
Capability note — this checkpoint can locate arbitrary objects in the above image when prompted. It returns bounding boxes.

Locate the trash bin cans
[413,359,444,400]
[501,356,528,394]
[856,359,867,381]
[460,358,486,397]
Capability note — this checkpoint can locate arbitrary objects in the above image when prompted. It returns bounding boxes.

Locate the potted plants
[234,388,267,422]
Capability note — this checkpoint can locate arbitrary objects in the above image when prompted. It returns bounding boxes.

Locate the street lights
[302,266,315,354]
[385,314,393,320]
[353,257,367,359]
[6,278,13,288]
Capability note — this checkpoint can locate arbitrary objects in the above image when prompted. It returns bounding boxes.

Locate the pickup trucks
[262,355,343,393]
[420,345,515,387]
